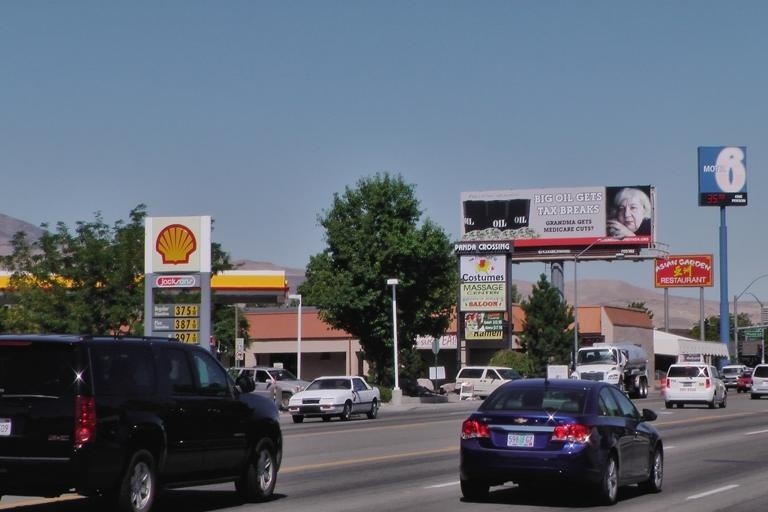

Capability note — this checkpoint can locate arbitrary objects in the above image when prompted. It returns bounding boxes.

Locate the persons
[604,187,652,240]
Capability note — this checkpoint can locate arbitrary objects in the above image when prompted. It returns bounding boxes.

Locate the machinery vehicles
[570,342,650,398]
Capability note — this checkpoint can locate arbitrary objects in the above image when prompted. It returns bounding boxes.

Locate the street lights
[385,277,401,387]
[732,273,766,364]
[570,238,605,365]
[288,291,306,380]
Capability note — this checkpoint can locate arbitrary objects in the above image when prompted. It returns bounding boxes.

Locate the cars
[286,374,383,424]
[663,361,768,412]
[456,377,650,511]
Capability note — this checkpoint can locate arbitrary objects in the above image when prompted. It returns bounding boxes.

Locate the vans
[456,365,523,399]
[225,364,317,412]
[0,333,283,510]
